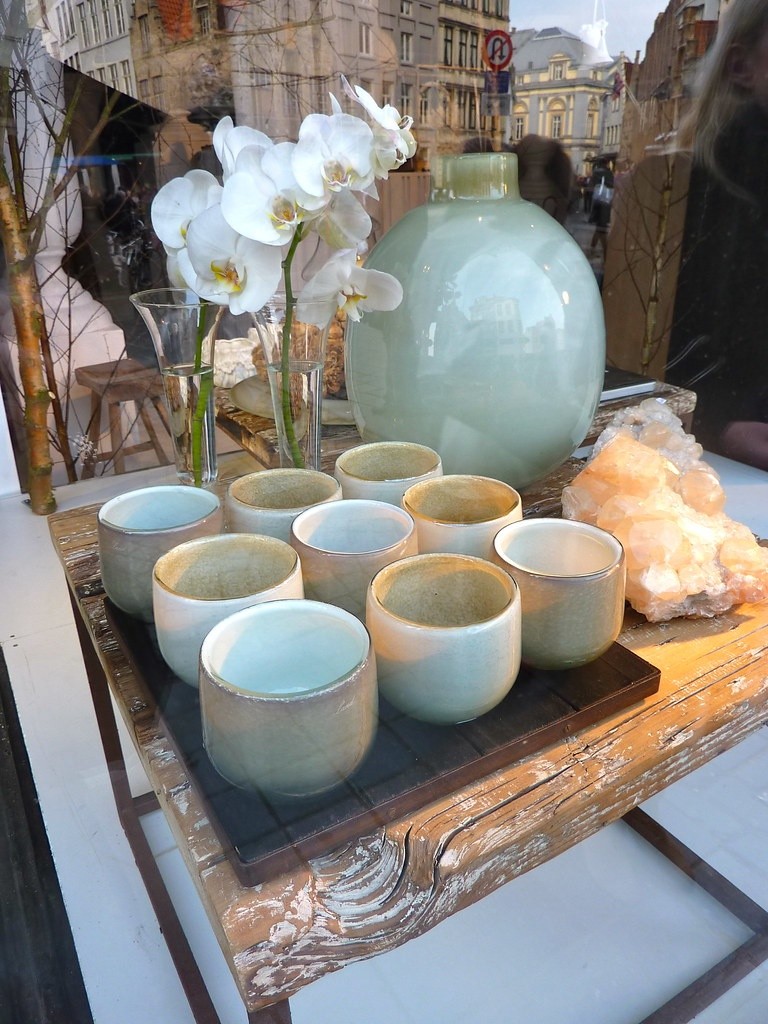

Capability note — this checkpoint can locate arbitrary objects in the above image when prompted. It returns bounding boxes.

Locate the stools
[75,360,169,480]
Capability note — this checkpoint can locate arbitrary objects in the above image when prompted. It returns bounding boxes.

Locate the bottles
[344,152,607,490]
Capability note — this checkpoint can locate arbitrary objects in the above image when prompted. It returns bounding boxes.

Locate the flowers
[144,72,420,493]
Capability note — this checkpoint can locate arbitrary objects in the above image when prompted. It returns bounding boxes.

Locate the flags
[612,70,624,100]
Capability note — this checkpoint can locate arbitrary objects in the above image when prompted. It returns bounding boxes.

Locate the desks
[153,363,698,469]
[46,430,768,1024]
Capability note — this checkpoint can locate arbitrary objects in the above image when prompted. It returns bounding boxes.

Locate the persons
[663,0,767,472]
[583,162,614,261]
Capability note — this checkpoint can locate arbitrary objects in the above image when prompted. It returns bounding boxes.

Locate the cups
[226,467,342,546]
[489,516,626,671]
[151,533,304,690]
[199,598,379,805]
[96,487,221,615]
[334,440,444,506]
[400,474,522,570]
[288,498,419,624]
[365,552,521,726]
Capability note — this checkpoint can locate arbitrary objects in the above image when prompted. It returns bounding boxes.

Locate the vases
[129,288,225,493]
[245,296,331,475]
